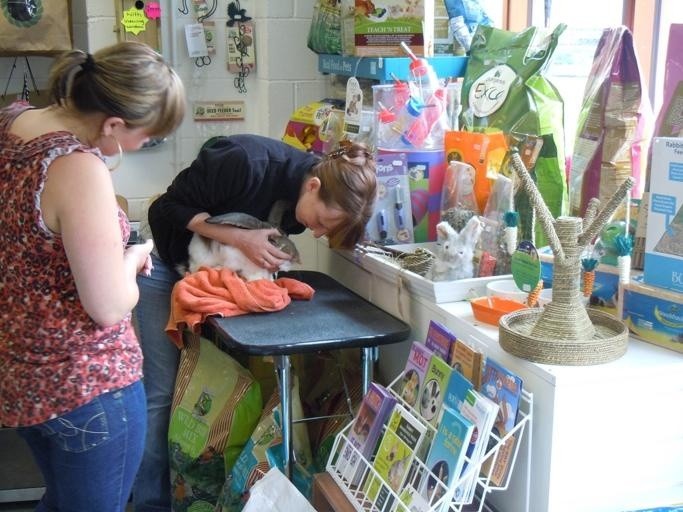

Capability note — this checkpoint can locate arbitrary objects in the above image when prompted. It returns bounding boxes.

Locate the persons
[0,38,185,511]
[128,132,378,510]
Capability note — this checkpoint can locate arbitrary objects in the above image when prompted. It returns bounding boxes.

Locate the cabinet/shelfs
[312,322,536,511]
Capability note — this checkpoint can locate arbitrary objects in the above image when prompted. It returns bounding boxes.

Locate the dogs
[421,380,439,418]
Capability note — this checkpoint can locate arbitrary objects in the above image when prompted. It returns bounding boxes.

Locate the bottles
[374,41,452,151]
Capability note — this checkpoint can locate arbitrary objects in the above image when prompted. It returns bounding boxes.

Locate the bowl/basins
[470,280,591,327]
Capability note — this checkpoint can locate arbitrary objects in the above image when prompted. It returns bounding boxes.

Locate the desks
[212,272,411,480]
[312,237,681,511]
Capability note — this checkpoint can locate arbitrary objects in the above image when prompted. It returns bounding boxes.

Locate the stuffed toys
[422,216,484,282]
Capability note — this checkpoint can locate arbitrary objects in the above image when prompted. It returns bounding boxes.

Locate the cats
[185,210,303,283]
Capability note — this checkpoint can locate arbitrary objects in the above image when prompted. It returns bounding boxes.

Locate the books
[335,310,534,510]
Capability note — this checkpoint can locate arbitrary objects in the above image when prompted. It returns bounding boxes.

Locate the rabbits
[386,447,412,486]
[400,371,419,404]
